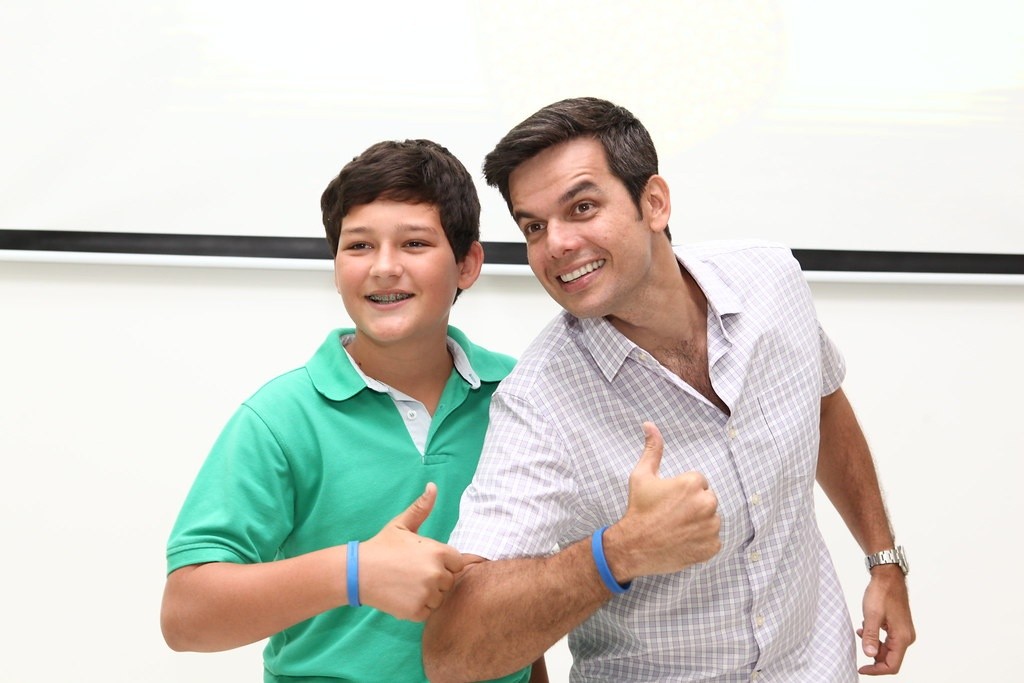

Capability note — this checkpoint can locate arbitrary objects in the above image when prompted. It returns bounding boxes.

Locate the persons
[160,138,548,683]
[420,83,917,683]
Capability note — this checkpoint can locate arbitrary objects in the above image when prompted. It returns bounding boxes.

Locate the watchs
[864,545,910,575]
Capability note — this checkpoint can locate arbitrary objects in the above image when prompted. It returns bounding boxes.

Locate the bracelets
[346,540,362,610]
[591,525,633,594]
